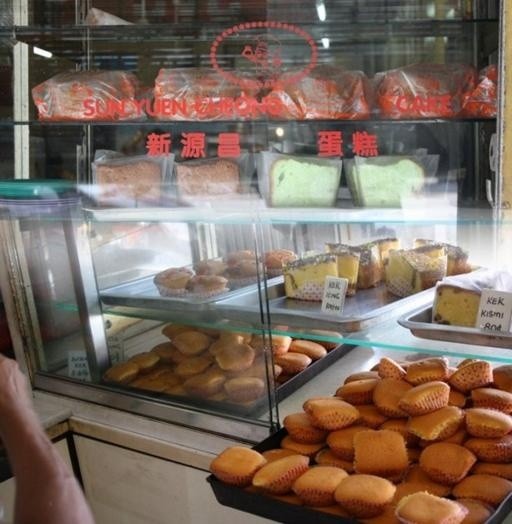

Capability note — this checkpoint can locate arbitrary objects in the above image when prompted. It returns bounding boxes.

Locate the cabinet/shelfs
[0,0,512,447]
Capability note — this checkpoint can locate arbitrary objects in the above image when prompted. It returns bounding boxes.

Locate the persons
[0,353,96,524]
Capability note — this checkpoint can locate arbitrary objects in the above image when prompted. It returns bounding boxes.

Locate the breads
[102,320,343,407]
[48,68,143,120]
[431,268,510,327]
[212,352,512,523]
[173,160,241,202]
[381,66,499,119]
[150,236,299,298]
[154,68,260,120]
[93,160,161,205]
[268,160,341,206]
[273,69,369,121]
[282,237,473,302]
[345,160,428,206]
